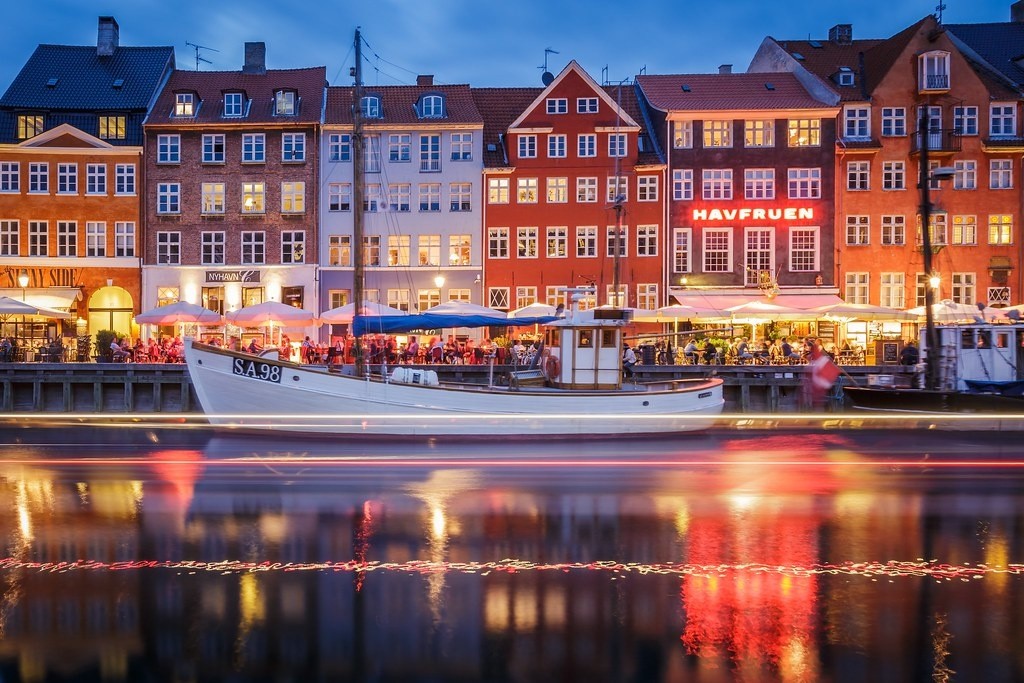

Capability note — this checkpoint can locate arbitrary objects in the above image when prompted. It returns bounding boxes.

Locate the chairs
[676,347,807,365]
[11,346,64,363]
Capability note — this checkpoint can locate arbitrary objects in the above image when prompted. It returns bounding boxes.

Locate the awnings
[0,288,84,308]
[669,285,850,324]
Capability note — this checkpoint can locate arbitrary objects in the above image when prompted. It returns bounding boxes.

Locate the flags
[808,342,841,391]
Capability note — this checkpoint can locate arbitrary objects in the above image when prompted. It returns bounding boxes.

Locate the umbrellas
[135,300,1024,366]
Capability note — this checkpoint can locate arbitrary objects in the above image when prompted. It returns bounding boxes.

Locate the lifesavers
[545,355,561,379]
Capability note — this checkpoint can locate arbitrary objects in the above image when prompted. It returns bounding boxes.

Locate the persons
[622,341,865,379]
[109,333,540,364]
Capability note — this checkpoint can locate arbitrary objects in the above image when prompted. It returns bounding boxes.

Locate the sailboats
[180,23,726,446]
[842,102,1024,411]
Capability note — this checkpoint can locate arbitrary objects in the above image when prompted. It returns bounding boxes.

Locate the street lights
[18,269,31,347]
[434,270,444,342]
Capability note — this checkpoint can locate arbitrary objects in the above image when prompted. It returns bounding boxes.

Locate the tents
[0,295,72,363]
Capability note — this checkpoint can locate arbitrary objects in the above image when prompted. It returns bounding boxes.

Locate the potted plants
[96,330,116,362]
